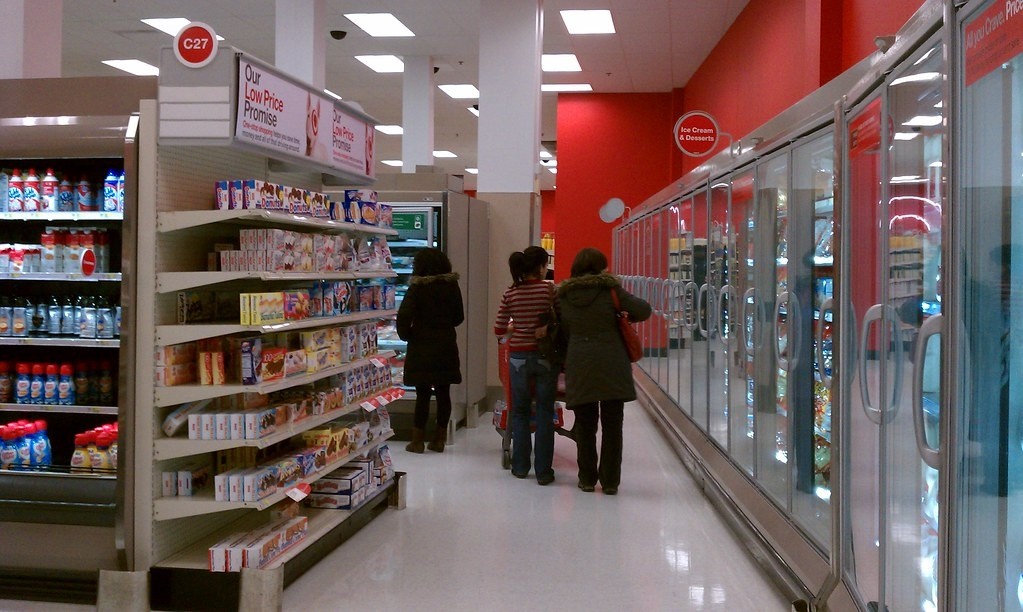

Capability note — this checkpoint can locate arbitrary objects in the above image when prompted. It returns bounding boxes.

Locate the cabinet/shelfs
[139,99,407,610]
[1,112,142,600]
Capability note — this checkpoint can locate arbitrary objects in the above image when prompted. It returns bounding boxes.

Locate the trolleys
[490,338,577,470]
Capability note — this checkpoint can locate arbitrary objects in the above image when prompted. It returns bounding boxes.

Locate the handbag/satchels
[609,287,643,362]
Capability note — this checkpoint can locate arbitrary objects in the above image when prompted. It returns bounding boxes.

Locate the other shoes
[511,468,526,478]
[538,475,555,485]
[601,483,617,495]
[578,480,595,492]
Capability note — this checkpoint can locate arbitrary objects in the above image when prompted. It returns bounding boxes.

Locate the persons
[557,247,652,495]
[493,245,562,485]
[396,246,464,454]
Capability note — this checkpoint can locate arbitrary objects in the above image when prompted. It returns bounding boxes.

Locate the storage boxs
[155,180,394,571]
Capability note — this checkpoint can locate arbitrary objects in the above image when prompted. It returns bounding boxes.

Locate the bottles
[541,233,555,269]
[195,337,262,385]
[0,164,126,477]
[493,400,564,430]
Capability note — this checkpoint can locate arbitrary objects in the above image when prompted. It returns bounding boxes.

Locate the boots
[427,424,448,453]
[406,427,426,454]
